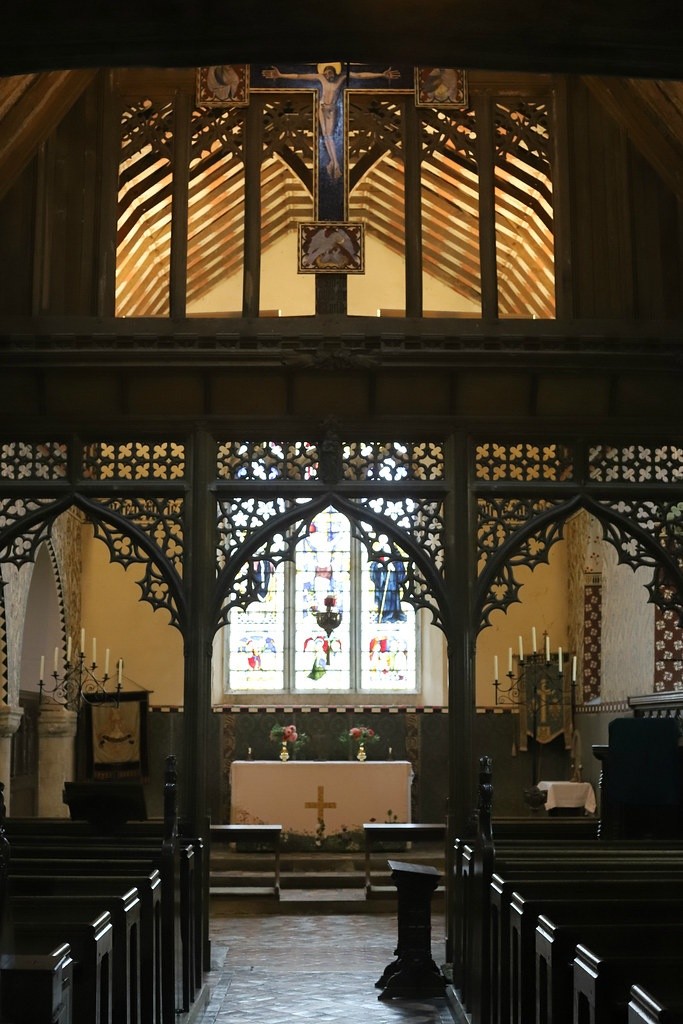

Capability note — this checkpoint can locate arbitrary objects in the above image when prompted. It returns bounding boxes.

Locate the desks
[208,823,283,900]
[537,780,597,816]
[229,759,415,855]
[363,822,449,899]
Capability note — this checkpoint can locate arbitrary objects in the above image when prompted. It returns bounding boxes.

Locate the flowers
[268,723,298,743]
[338,725,381,745]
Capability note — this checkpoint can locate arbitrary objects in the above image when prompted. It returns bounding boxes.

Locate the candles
[93,637,97,663]
[572,656,577,682]
[546,636,550,661]
[66,635,72,661]
[118,659,123,684]
[40,656,44,680]
[509,647,512,672]
[53,646,58,671]
[519,635,523,660]
[494,655,498,680]
[81,627,85,652]
[105,649,109,673]
[558,647,563,673]
[531,626,536,652]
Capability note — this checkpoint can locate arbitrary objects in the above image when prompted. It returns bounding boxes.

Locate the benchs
[0,814,212,1024]
[442,812,683,1024]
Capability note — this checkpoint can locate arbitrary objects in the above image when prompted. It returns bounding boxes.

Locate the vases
[279,740,289,761]
[356,743,367,761]
